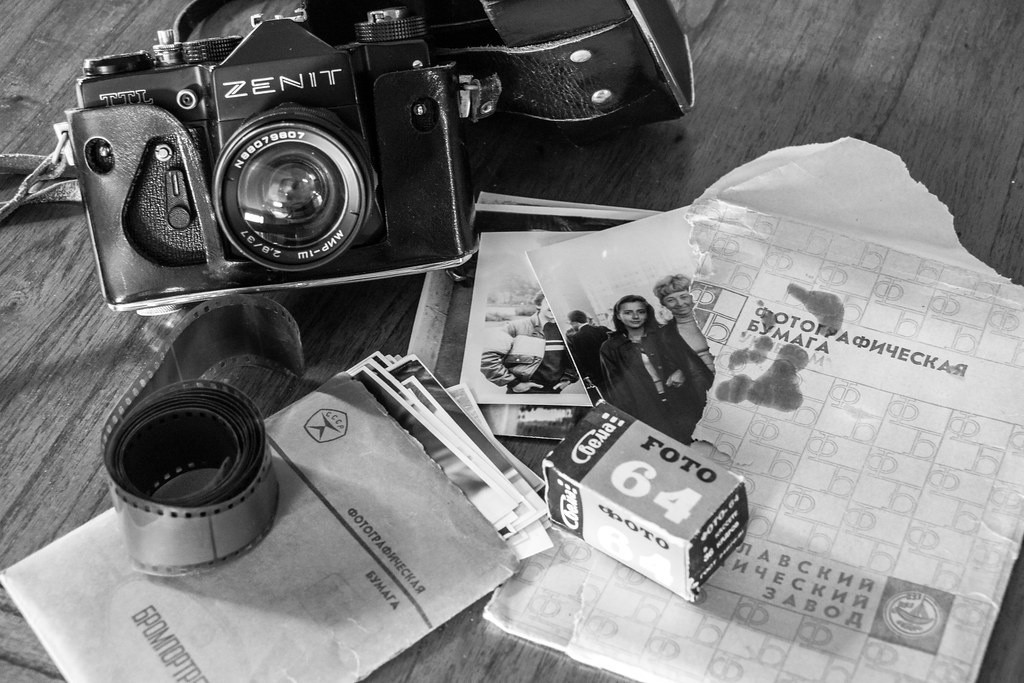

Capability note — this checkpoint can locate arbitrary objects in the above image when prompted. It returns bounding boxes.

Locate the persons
[480,291,579,394]
[650,275,716,411]
[565,310,614,404]
[602,295,699,444]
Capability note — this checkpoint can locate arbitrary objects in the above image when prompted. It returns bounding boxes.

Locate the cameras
[76,13,477,313]
